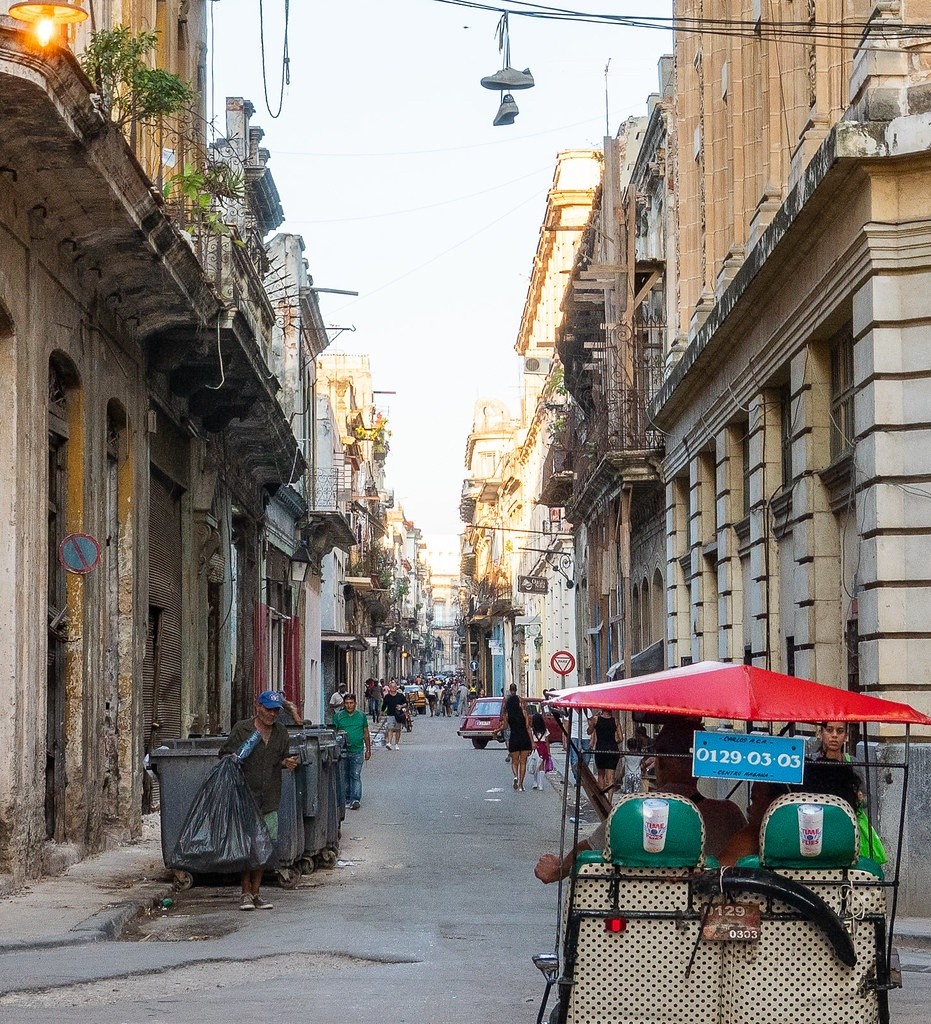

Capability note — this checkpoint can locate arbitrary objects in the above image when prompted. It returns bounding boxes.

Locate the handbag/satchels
[381,702,387,712]
[856,803,888,865]
[526,748,543,774]
[545,756,552,773]
[450,694,456,703]
[395,710,405,723]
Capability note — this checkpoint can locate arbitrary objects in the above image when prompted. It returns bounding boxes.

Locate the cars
[397,685,427,715]
[456,697,562,749]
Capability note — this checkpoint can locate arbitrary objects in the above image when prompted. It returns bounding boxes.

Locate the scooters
[531,660,931,1024]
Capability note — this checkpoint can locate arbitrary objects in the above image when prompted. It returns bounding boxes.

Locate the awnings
[605,636,665,683]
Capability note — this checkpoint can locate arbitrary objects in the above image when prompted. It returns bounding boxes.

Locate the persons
[328,672,485,724]
[633,725,658,764]
[531,715,747,887]
[527,712,551,791]
[491,694,536,792]
[542,688,566,713]
[380,683,409,752]
[804,719,863,810]
[586,709,624,804]
[614,737,643,795]
[331,693,372,811]
[276,689,304,726]
[716,756,863,873]
[501,683,525,761]
[561,704,596,786]
[217,690,300,911]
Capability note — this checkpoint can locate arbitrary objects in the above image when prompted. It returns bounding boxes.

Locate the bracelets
[366,750,371,753]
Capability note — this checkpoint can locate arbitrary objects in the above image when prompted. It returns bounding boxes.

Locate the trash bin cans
[148,723,350,890]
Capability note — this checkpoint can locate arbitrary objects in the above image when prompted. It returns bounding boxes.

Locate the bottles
[235,729,263,760]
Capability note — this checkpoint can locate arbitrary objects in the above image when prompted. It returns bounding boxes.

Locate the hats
[459,684,465,686]
[258,691,284,710]
[641,721,707,753]
[373,678,378,681]
[444,685,448,687]
[339,683,345,686]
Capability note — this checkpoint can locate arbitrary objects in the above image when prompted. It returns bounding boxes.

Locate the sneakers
[253,895,273,909]
[239,893,255,910]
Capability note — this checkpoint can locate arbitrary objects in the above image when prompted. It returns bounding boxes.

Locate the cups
[796,804,824,857]
[642,798,670,853]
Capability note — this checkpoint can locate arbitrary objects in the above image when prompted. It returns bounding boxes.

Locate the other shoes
[532,784,538,788]
[394,745,399,750]
[386,744,392,750]
[537,785,543,790]
[350,801,360,810]
[513,777,518,790]
[519,788,525,791]
[346,804,350,808]
